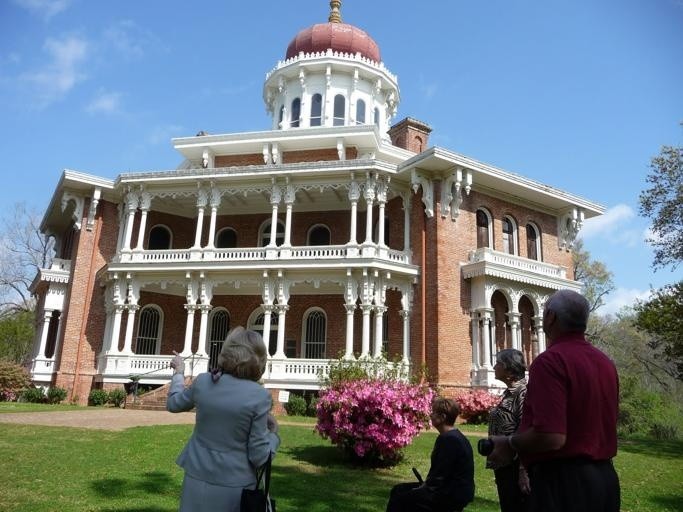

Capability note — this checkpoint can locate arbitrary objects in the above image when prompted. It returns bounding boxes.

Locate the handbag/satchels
[239,488,276,512]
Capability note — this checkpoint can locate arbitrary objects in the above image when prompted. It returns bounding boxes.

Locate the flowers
[315,350,438,470]
[456,391,502,424]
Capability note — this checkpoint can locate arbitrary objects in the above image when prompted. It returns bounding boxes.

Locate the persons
[489,289,620,512]
[165,326,281,512]
[486,348,531,512]
[385,398,475,512]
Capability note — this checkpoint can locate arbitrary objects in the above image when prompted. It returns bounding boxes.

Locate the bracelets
[508,435,513,448]
